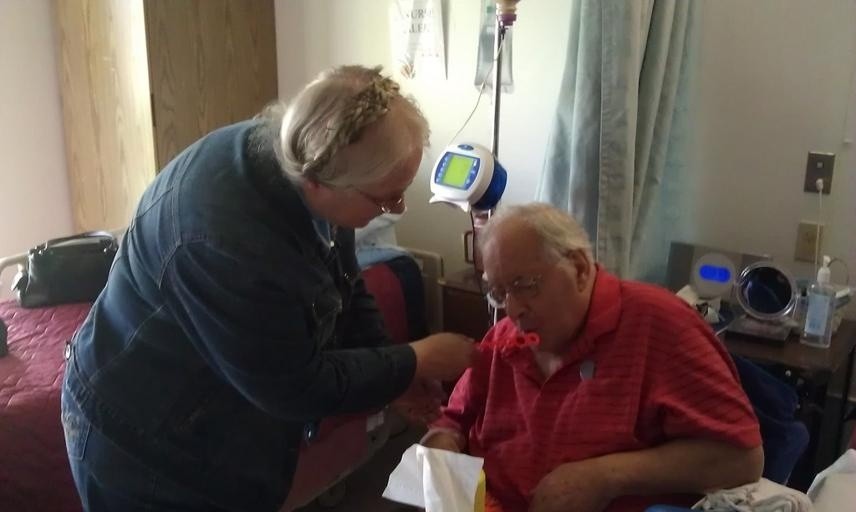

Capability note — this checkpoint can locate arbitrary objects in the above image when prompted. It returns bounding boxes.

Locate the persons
[60,66,475,510]
[416,202,765,512]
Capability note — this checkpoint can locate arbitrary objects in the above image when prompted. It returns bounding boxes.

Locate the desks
[711,299,856,493]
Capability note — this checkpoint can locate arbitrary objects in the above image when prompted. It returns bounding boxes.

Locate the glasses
[486,250,570,309]
[347,183,405,215]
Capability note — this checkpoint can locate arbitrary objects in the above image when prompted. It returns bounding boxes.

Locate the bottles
[801,267,832,349]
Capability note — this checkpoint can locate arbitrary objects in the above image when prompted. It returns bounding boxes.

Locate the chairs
[648,351,810,511]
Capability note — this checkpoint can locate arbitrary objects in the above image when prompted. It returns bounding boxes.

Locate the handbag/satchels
[11,230,120,309]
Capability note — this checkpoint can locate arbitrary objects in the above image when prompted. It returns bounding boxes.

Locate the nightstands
[438,267,506,404]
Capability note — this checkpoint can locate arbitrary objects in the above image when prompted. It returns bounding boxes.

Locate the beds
[1,222,443,511]
